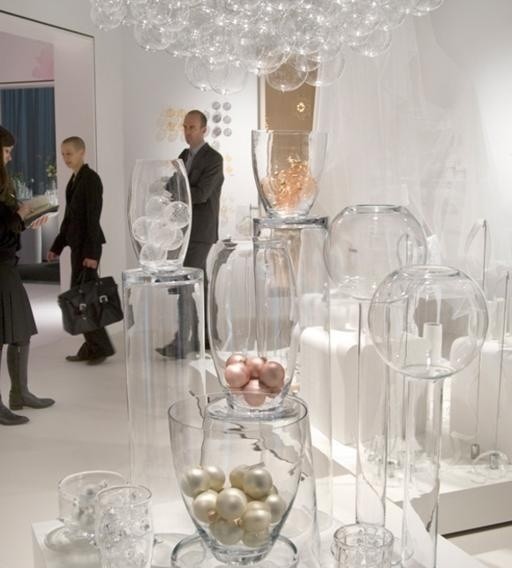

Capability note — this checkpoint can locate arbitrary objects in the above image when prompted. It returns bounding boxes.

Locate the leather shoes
[155,335,222,359]
[66,341,115,366]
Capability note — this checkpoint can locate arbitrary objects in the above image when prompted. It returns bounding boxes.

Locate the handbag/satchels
[58,266,123,336]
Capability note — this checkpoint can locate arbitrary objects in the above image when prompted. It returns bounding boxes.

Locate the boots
[0,343,30,426]
[6,343,55,410]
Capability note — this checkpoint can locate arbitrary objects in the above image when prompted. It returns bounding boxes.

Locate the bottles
[124,157,194,279]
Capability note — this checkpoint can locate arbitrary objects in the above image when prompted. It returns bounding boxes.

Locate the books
[17,189,61,229]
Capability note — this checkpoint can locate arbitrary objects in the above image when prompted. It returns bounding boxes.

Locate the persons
[154,109,224,359]
[1,126,56,426]
[46,136,116,366]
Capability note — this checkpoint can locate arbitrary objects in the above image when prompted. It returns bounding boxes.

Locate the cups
[169,392,307,567]
[57,471,153,568]
[251,127,328,222]
[203,237,302,422]
[123,272,200,435]
[333,526,395,567]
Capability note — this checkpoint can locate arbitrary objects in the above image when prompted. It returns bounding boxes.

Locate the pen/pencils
[8,192,34,213]
